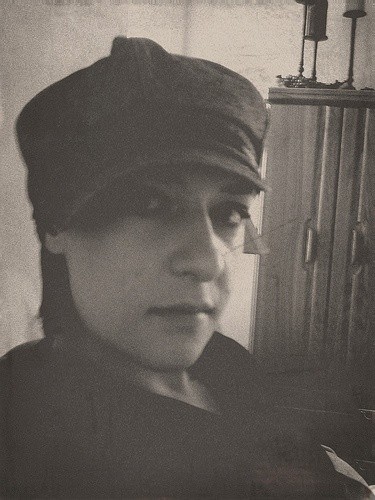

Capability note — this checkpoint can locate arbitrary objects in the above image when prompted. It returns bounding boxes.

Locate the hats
[14,35,274,237]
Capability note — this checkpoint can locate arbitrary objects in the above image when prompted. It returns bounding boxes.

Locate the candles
[346,0,364,12]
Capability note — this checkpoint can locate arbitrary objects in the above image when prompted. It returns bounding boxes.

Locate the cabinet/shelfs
[248,86,375,414]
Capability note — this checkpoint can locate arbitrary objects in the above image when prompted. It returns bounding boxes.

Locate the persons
[0,35,375,500]
